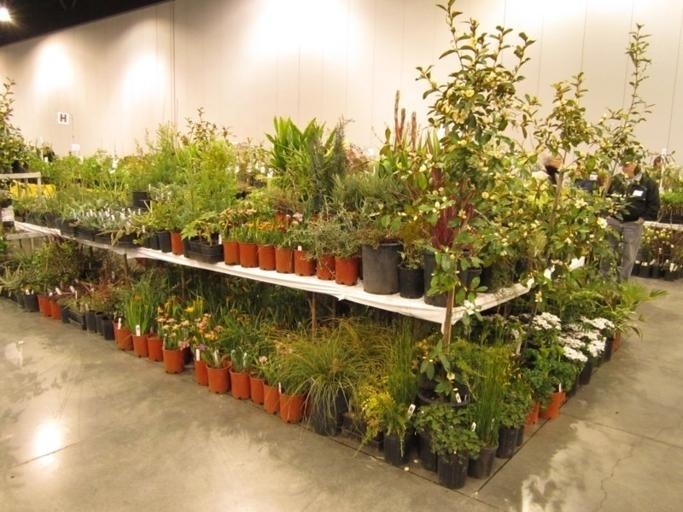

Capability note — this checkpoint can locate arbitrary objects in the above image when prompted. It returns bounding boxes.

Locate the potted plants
[0,0,683,494]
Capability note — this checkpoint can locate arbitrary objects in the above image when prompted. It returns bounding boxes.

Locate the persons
[575,156,595,194]
[600,155,660,283]
[536,150,562,193]
[654,157,662,167]
